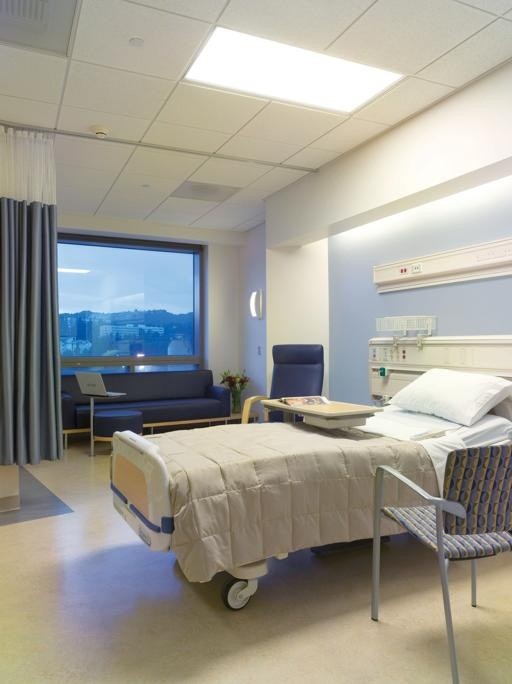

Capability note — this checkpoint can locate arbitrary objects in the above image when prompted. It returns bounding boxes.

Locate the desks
[262,400,384,429]
[80,395,127,456]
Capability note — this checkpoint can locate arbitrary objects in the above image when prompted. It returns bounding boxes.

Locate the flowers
[219,368,255,412]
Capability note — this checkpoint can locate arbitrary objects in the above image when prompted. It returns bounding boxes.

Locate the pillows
[385,368,512,426]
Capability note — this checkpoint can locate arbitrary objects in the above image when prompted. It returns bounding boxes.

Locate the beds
[110,403,512,609]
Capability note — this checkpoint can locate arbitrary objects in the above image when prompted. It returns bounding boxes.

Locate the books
[280,395,333,407]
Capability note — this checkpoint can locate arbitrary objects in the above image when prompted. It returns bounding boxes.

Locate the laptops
[75,372,127,397]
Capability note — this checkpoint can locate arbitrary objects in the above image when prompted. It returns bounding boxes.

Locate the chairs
[241,344,324,424]
[370,445,512,684]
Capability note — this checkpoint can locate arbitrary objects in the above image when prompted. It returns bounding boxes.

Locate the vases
[232,391,241,413]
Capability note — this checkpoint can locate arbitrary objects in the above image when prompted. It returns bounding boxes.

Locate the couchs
[61,370,230,448]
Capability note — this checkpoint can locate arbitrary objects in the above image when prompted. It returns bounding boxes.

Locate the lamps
[250,289,262,355]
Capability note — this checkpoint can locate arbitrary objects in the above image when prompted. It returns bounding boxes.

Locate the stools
[94,411,143,441]
